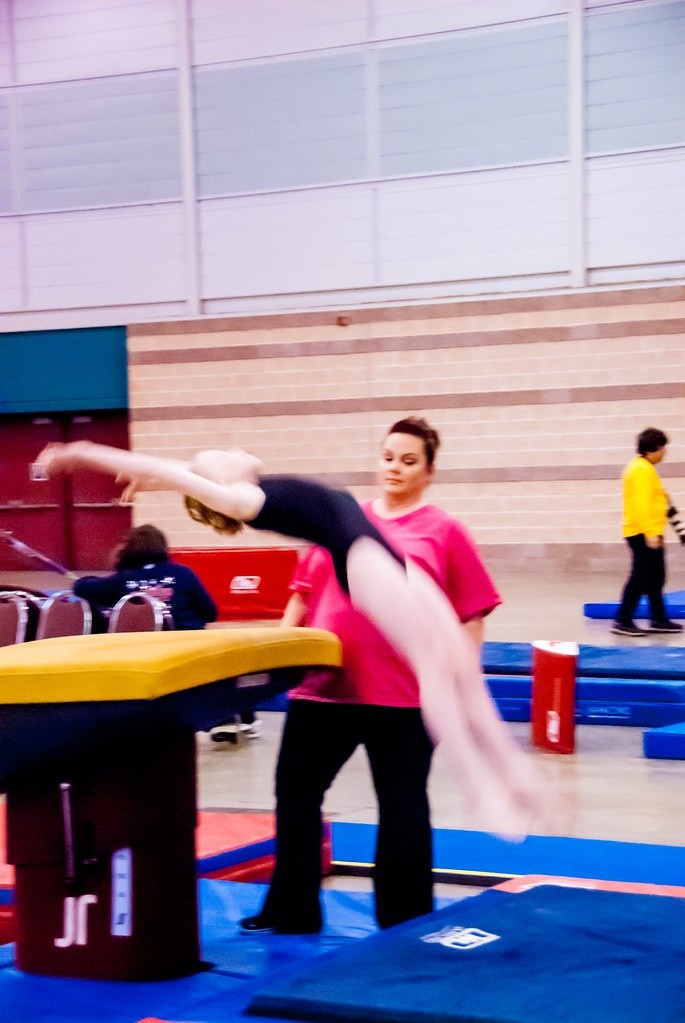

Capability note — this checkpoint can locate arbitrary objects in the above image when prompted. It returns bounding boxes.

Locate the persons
[609,428,685,637]
[72,526,261,744]
[238,416,502,936]
[38,441,570,842]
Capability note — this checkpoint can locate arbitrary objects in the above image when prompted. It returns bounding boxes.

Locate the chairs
[0,590,174,646]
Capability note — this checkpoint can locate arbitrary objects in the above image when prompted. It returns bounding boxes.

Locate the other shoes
[241,915,271,931]
[650,618,683,632]
[210,718,262,742]
[612,621,648,636]
